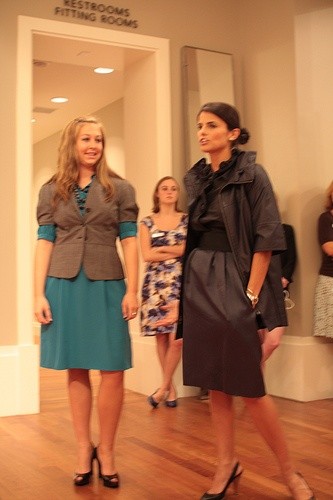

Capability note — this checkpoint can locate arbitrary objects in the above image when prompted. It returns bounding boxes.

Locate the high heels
[288,472,314,500]
[148,387,163,407]
[199,460,245,500]
[164,392,176,407]
[71,441,120,488]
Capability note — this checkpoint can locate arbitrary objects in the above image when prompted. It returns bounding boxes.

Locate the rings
[132,312,137,316]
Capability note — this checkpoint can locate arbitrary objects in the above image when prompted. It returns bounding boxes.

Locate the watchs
[246,291,259,306]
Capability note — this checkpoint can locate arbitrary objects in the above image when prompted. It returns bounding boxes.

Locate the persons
[32,116,141,488]
[314,181,333,341]
[182,101,317,500]
[253,223,298,363]
[139,175,190,408]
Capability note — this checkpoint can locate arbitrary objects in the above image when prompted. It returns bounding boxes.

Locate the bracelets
[247,287,258,297]
